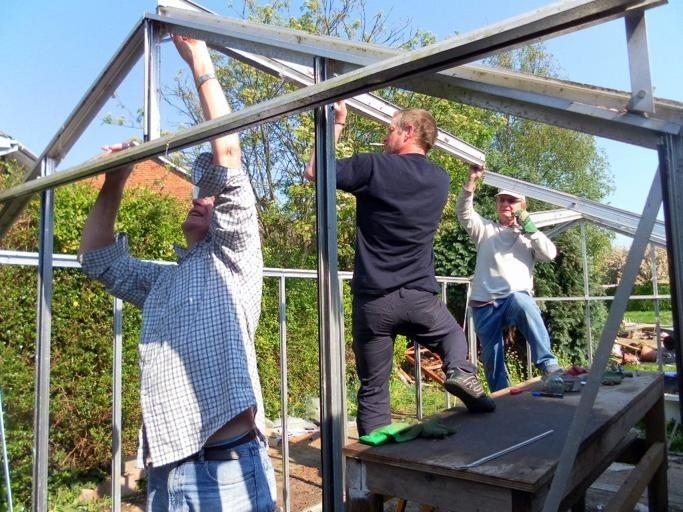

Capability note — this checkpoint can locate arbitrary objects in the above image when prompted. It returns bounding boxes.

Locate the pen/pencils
[532,391,564,397]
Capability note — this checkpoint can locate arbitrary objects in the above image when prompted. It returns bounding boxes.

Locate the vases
[621,352,638,364]
[637,345,656,362]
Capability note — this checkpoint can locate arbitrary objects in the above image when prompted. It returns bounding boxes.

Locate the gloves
[514,208,537,234]
[358,417,423,447]
[417,414,456,439]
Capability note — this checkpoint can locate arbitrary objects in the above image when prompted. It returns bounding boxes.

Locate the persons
[78,32,277,512]
[454,163,562,394]
[303,72,495,440]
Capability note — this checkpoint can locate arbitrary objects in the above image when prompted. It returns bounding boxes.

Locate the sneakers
[358,488,383,512]
[442,367,496,410]
[541,364,581,392]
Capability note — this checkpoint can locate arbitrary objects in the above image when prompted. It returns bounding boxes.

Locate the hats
[494,188,525,201]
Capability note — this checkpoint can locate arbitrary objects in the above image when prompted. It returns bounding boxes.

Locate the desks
[343,367,670,512]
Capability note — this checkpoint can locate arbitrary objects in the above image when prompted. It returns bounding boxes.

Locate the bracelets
[335,122,345,126]
[195,71,218,92]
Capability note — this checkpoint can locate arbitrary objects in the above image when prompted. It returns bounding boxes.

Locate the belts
[183,427,259,462]
